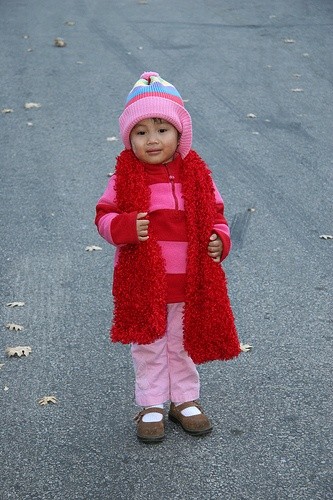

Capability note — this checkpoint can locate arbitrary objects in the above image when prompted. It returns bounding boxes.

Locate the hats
[118,70,193,159]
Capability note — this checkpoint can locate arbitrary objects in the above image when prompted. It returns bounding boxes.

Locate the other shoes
[133,406,165,444]
[168,401,213,436]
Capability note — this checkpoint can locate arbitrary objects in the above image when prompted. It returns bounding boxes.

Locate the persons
[95,72,231,442]
